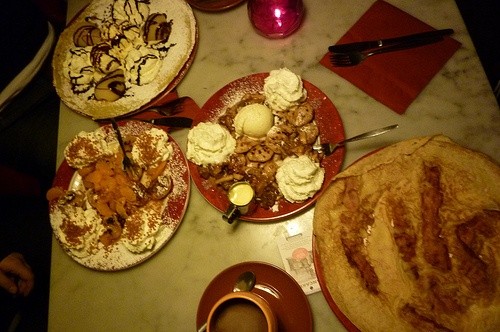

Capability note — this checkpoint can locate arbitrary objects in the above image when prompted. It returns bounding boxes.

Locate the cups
[206,292,278,332]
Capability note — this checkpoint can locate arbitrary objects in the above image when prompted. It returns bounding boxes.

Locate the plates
[186,72,346,223]
[53,0,199,119]
[196,261,312,332]
[312,145,500,332]
[186,0,244,12]
[48,120,191,271]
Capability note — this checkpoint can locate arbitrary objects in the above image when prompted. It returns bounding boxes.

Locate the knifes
[328,28,455,54]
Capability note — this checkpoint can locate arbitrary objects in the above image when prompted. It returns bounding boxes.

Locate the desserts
[62,0,191,121]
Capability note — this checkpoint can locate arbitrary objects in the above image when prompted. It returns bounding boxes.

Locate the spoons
[198,271,257,332]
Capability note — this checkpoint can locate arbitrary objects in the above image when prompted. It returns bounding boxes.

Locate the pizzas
[313,133,500,332]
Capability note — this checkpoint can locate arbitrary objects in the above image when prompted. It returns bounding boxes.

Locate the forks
[111,120,136,179]
[329,37,443,67]
[312,124,400,157]
[146,99,184,116]
